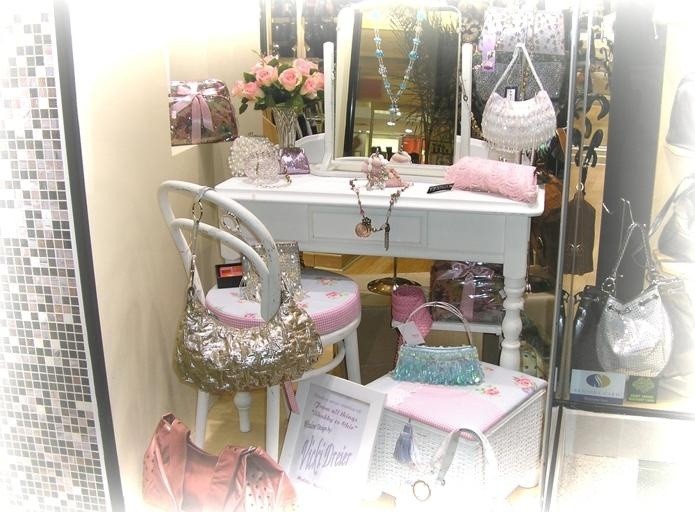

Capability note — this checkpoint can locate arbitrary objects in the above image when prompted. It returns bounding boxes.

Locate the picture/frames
[279,373,388,507]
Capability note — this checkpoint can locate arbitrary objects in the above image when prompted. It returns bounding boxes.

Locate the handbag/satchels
[480,5,566,57]
[241,240,304,301]
[172,184,324,394]
[529,192,598,276]
[139,411,298,511]
[663,76,695,149]
[274,148,310,178]
[571,220,694,379]
[169,79,239,147]
[430,262,551,379]
[446,156,539,204]
[482,42,557,154]
[596,222,675,378]
[473,62,565,100]
[659,188,695,261]
[393,299,485,386]
[394,428,504,510]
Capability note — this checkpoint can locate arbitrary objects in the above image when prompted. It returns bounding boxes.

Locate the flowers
[228,47,330,117]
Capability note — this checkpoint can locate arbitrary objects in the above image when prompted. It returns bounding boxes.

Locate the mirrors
[322,0,470,179]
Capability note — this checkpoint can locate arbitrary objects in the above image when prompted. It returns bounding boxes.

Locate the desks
[213,164,546,434]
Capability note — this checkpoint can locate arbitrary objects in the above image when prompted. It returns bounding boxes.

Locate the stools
[366,359,548,499]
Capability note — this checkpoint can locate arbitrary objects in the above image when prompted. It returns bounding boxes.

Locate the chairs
[156,180,361,464]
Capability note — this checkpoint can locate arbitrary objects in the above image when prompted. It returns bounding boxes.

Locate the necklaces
[372,7,426,126]
[366,151,390,193]
[347,178,413,253]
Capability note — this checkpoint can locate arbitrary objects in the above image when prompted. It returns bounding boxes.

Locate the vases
[270,102,300,148]
